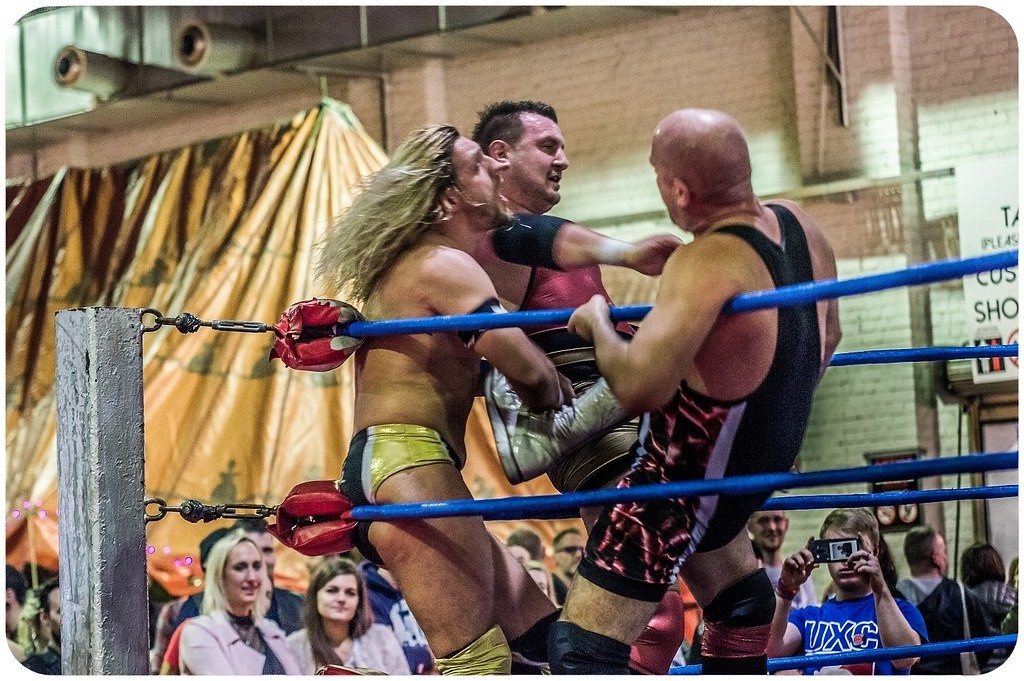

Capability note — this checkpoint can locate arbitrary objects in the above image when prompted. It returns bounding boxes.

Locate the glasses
[558,545,586,553]
[752,517,785,526]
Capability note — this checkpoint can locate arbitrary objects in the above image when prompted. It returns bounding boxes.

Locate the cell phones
[805,535,861,564]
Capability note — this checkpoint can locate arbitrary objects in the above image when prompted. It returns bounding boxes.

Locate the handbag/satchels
[959,646,981,675]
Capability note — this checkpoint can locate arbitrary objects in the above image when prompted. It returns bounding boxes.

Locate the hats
[199,527,232,571]
[507,530,540,561]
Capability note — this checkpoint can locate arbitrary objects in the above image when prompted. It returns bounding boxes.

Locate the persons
[469,100,777,673]
[313,125,579,677]
[5,505,1019,677]
[482,105,843,673]
